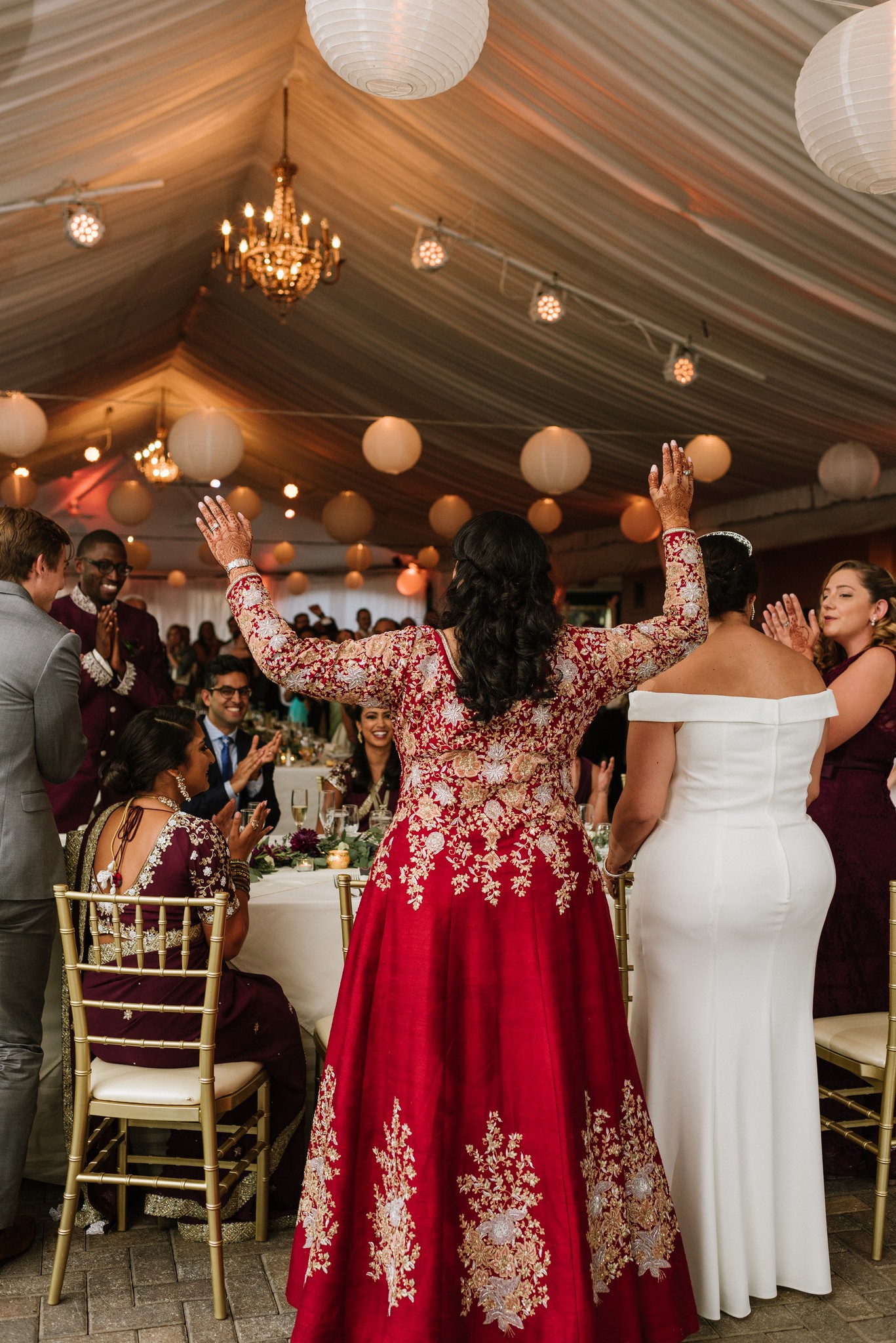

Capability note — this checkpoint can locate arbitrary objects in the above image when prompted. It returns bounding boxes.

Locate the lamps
[207,84,348,330]
[662,336,700,388]
[58,198,110,251]
[132,387,190,493]
[410,215,453,274]
[525,270,566,327]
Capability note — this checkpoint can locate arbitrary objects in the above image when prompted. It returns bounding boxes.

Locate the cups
[241,809,256,833]
[342,804,358,835]
[326,809,349,837]
[327,850,350,868]
[577,803,593,834]
[249,802,265,829]
[369,810,392,833]
[296,857,314,871]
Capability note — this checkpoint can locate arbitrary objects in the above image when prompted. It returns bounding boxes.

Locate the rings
[210,522,220,531]
[783,621,790,627]
[269,751,272,755]
[683,469,691,476]
[248,819,260,830]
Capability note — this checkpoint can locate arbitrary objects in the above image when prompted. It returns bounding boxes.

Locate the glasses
[207,684,252,700]
[79,555,133,578]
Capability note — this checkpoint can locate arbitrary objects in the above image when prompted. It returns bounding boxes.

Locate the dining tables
[223,723,368,839]
[213,828,646,1088]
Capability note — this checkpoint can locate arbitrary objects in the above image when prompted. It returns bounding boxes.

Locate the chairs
[308,865,371,1128]
[810,876,896,1266]
[46,881,274,1321]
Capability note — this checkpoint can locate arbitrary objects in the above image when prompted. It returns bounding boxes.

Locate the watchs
[226,557,255,578]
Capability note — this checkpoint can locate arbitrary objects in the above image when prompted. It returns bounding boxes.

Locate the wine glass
[319,790,337,837]
[291,788,308,831]
[594,824,612,862]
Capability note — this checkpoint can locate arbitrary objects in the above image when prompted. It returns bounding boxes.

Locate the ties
[219,734,234,785]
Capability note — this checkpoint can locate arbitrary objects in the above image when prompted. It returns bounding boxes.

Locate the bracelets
[603,855,633,878]
[229,858,252,902]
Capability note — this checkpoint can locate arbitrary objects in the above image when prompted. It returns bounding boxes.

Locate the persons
[761,559,896,1174]
[0,502,91,1264]
[571,742,622,832]
[314,703,403,837]
[592,530,837,1324]
[60,702,331,1243]
[40,528,170,833]
[179,654,284,835]
[119,590,662,736]
[194,438,710,1343]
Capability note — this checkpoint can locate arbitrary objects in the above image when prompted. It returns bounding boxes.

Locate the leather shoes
[0,1215,36,1270]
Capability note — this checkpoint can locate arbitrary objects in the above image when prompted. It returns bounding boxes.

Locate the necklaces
[110,794,182,871]
